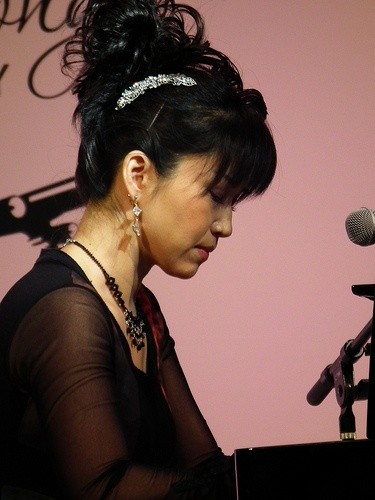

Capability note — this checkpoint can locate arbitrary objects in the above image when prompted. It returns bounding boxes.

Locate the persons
[0,1,278,500]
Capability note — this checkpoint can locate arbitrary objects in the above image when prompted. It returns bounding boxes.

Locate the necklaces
[64,238,147,349]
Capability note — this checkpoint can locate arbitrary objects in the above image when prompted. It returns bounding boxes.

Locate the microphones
[345,207,375,246]
